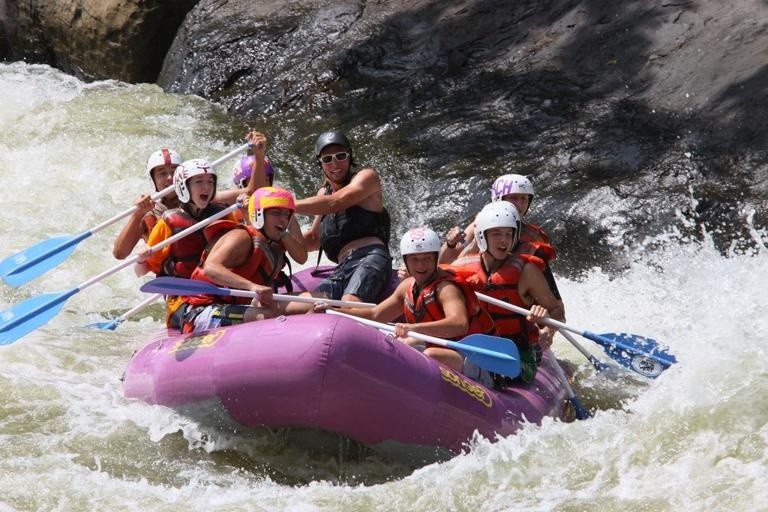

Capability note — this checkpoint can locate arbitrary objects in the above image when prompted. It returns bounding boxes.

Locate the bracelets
[136,260,145,266]
[551,326,556,337]
[446,240,458,249]
[280,229,289,241]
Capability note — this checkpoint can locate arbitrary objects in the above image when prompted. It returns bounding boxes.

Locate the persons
[312,227,470,376]
[437,173,555,264]
[236,127,391,318]
[435,201,566,354]
[110,128,269,259]
[397,201,565,387]
[230,155,308,265]
[134,157,251,331]
[177,186,317,334]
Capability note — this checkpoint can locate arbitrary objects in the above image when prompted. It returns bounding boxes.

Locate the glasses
[318,151,350,165]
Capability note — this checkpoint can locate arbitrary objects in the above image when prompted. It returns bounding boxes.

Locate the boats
[120,263,568,462]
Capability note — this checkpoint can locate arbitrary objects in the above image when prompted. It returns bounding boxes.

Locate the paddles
[0,203,241,344]
[326,310,521,377]
[138,275,377,309]
[476,293,678,378]
[80,292,163,332]
[0,142,254,289]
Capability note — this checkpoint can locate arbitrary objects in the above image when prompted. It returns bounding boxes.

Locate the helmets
[248,186,296,230]
[473,200,522,253]
[232,154,274,189]
[399,227,442,275]
[490,173,535,216]
[146,148,184,192]
[172,158,218,204]
[315,130,352,157]
